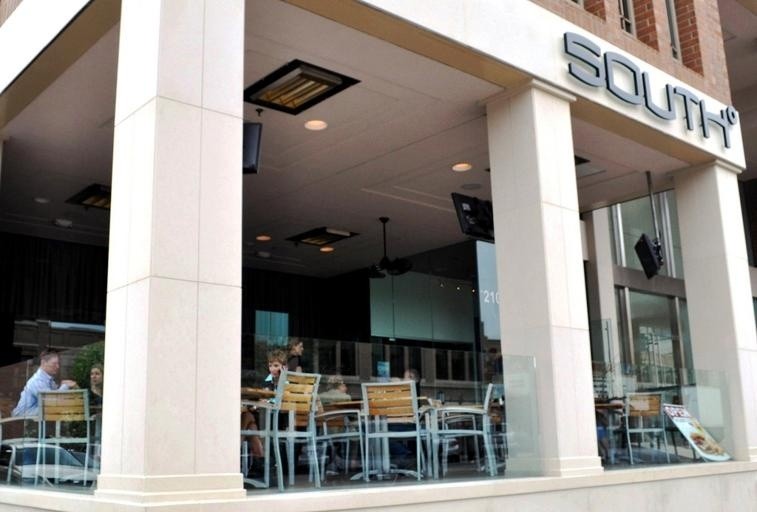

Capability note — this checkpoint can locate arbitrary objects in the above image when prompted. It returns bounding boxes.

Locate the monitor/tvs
[449,190,494,245]
[242,119,264,176]
[633,232,663,281]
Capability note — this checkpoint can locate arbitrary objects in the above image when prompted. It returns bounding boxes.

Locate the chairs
[594,367,673,466]
[241,366,505,492]
[0,387,101,496]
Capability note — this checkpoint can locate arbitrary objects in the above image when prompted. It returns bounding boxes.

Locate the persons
[11,349,81,420]
[318,371,352,400]
[285,336,304,373]
[402,369,421,409]
[259,349,288,390]
[241,402,265,472]
[88,365,102,406]
